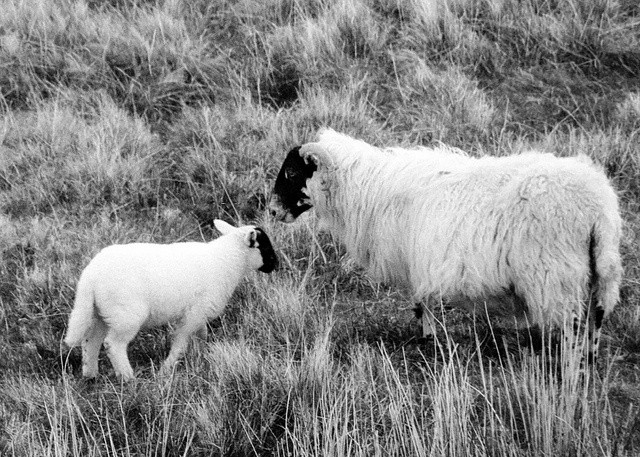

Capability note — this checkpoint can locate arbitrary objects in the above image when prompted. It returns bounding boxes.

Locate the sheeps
[268,125,624,385]
[65,218,279,389]
